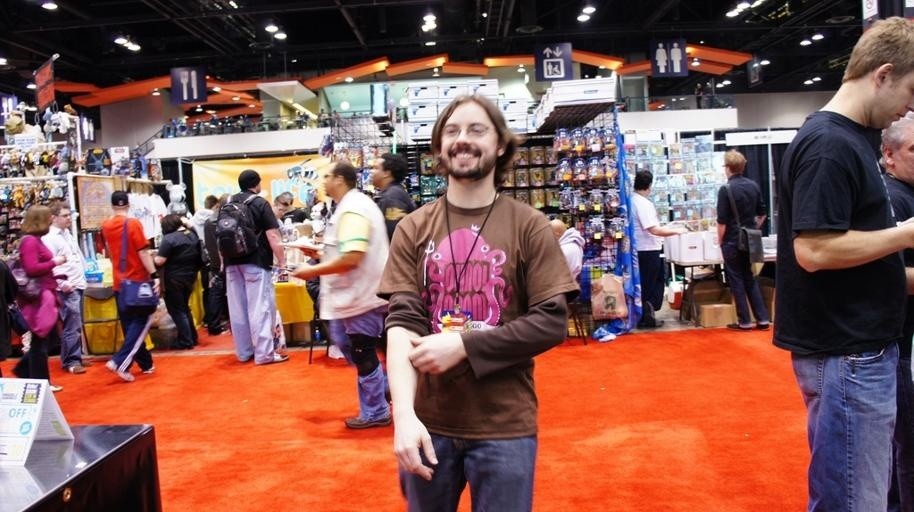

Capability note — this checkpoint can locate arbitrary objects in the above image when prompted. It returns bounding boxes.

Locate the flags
[32,62,54,112]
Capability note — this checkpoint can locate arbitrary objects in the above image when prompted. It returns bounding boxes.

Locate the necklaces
[444,191,498,317]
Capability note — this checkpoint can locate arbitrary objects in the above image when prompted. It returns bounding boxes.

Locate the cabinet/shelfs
[0,140,70,189]
[0,422,162,512]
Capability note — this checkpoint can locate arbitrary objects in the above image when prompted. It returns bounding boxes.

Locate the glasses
[441,125,497,140]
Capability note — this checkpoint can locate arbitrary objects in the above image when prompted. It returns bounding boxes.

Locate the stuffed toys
[41,107,56,133]
[50,112,70,134]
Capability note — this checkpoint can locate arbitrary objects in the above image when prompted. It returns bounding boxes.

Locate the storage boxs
[662,213,778,332]
[401,76,616,142]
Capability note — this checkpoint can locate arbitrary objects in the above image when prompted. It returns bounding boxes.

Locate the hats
[112,190,128,206]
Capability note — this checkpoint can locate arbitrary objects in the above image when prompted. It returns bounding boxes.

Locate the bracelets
[151,271,160,279]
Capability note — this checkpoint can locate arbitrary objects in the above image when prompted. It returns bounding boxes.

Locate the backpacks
[215,193,265,259]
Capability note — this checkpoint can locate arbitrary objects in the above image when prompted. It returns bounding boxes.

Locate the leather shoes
[638,320,664,329]
[757,324,770,331]
[727,323,752,331]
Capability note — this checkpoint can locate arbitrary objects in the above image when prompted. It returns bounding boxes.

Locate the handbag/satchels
[117,278,159,316]
[7,305,30,337]
[737,226,765,265]
[7,236,41,300]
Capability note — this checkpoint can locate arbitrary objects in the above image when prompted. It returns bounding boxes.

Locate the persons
[368,152,417,237]
[375,92,582,512]
[217,169,308,365]
[773,14,914,512]
[160,109,361,138]
[288,159,391,430]
[549,213,585,302]
[1,200,88,391]
[717,149,773,331]
[101,191,161,381]
[878,114,914,512]
[630,168,685,329]
[154,192,232,348]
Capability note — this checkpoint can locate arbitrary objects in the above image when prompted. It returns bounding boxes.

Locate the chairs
[566,275,589,347]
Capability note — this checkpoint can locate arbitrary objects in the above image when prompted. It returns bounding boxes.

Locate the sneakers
[50,383,63,392]
[344,413,392,429]
[273,351,289,363]
[105,360,135,382]
[67,365,86,374]
[202,317,230,336]
[140,364,155,374]
